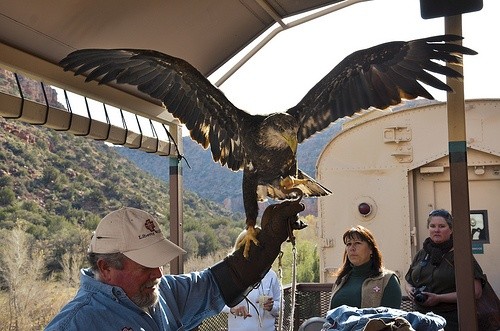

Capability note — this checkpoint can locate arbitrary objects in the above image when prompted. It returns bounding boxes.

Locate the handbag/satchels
[474,280,500,331]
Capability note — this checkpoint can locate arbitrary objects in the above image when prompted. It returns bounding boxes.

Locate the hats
[87,207,186,268]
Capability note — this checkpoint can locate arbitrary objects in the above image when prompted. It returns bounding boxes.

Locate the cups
[258,289,272,308]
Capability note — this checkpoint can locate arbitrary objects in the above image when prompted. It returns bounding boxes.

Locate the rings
[239,312,241,316]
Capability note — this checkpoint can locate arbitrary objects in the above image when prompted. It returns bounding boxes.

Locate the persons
[44,201,307,331]
[404,208,483,331]
[221,225,280,331]
[328,225,402,325]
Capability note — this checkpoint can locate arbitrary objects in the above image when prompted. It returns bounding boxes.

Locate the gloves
[210,201,306,307]
